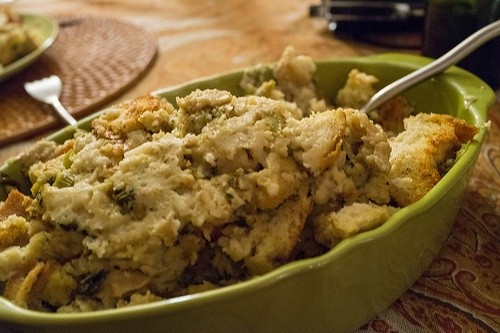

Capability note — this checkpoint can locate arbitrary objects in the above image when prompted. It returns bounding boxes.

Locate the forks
[22,71,77,127]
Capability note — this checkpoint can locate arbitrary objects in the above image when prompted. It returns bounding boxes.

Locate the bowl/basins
[0,52,498,333]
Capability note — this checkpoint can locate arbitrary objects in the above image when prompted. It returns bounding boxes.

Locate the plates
[0,12,60,80]
